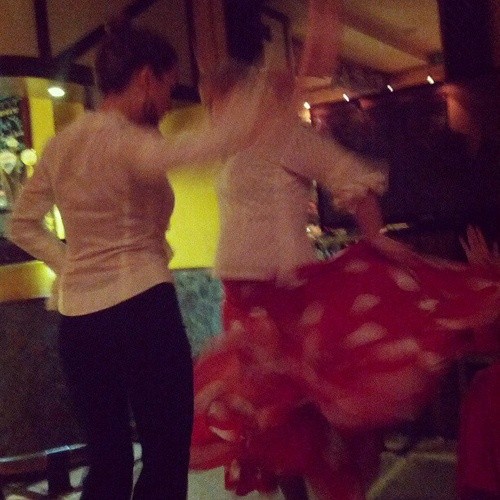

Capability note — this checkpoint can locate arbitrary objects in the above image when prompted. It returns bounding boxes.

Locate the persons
[212,57,390,500]
[4,21,299,500]
